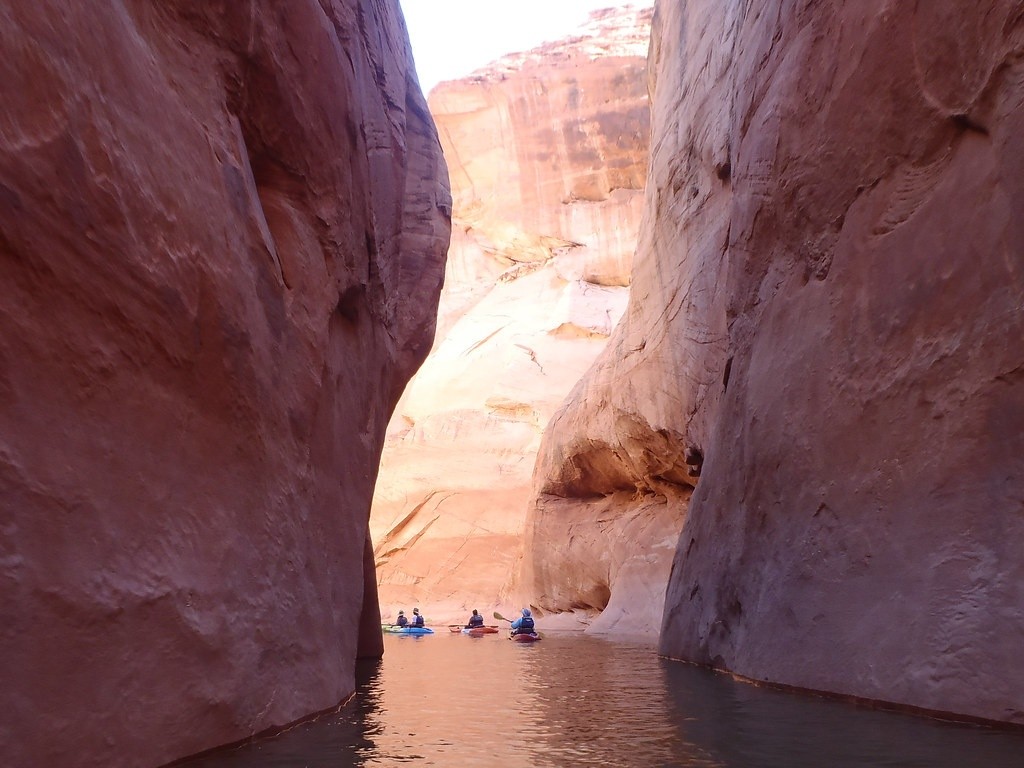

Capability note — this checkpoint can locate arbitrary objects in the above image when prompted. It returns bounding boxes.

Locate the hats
[522,608,531,616]
[397,610,405,616]
[412,608,419,614]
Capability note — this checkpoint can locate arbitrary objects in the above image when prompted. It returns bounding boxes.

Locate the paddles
[381,623,412,629]
[446,624,499,628]
[492,611,546,638]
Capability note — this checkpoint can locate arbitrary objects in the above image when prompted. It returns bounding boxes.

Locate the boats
[382,624,434,634]
[512,632,542,643]
[449,625,500,634]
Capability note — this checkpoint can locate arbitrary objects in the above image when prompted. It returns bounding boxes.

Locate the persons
[411,608,425,626]
[389,610,408,628]
[511,608,535,631]
[468,609,483,625]
